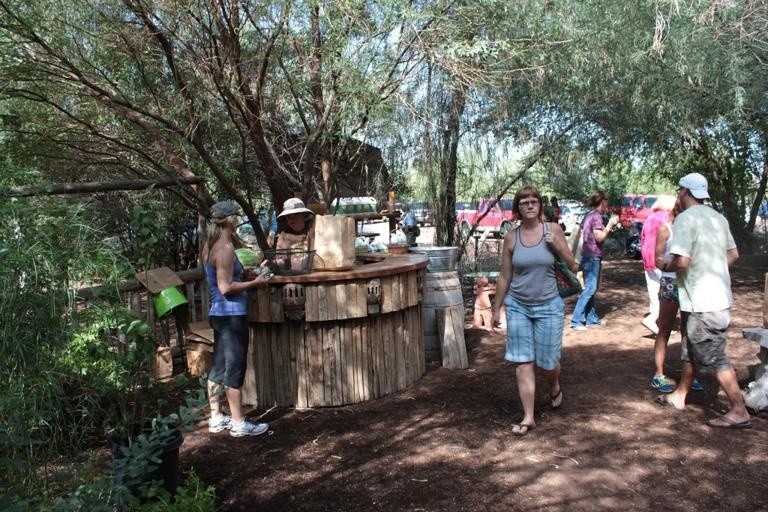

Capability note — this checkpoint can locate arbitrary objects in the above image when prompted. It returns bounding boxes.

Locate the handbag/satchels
[310,213,357,272]
[546,222,583,299]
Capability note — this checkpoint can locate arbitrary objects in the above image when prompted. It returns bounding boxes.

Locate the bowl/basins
[410,246,458,269]
[464,271,501,282]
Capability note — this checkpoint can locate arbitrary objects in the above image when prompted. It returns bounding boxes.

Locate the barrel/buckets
[422,272,465,362]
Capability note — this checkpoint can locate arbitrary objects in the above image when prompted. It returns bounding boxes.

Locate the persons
[639,192,678,337]
[540,196,566,231]
[655,171,755,431]
[489,185,581,433]
[273,196,318,270]
[648,198,704,394]
[550,197,570,214]
[569,190,622,332]
[400,205,416,244]
[200,200,273,439]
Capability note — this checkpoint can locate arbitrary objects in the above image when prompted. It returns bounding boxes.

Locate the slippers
[549,385,564,409]
[706,418,752,429]
[510,420,537,436]
[653,394,689,412]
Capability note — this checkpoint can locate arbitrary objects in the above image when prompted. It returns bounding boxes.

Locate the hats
[209,200,243,219]
[275,197,316,222]
[678,172,711,200]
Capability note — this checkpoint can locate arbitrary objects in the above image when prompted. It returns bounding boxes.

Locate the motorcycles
[623,222,642,260]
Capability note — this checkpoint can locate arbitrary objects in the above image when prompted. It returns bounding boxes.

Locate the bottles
[332,197,371,215]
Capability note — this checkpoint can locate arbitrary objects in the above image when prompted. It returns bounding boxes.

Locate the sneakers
[690,377,704,391]
[570,317,678,335]
[650,375,673,393]
[229,417,270,438]
[208,412,232,433]
